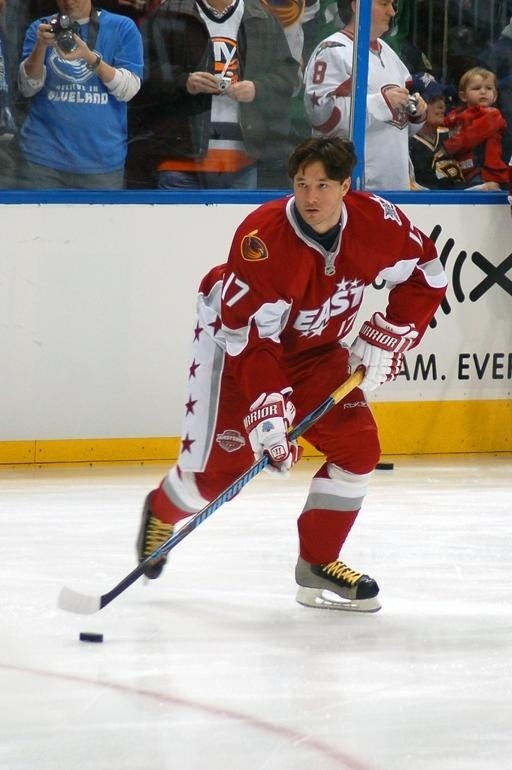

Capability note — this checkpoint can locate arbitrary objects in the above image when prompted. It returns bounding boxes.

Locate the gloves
[244,393,304,477]
[346,312,418,393]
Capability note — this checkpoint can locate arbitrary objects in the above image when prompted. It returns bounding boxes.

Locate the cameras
[217,77,233,92]
[46,13,80,54]
[399,95,419,117]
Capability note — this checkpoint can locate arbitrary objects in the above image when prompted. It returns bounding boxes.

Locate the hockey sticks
[57,367,363,614]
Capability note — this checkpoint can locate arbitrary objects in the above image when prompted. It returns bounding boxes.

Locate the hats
[409,70,445,102]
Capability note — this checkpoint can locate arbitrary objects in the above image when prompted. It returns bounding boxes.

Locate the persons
[136,134,448,600]
[0,2,512,193]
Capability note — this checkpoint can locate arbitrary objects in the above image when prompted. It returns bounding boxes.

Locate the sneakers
[295,556,379,600]
[136,492,176,581]
